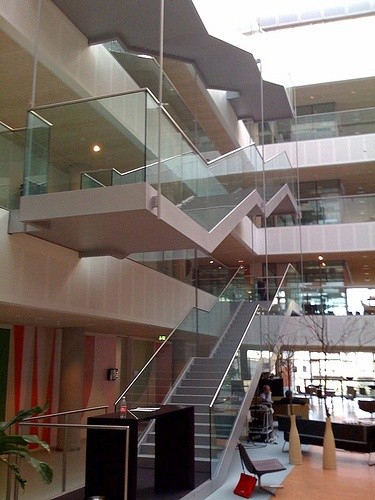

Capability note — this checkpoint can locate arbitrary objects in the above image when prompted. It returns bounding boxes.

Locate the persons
[257,281,267,301]
[279,390,306,405]
[260,385,273,403]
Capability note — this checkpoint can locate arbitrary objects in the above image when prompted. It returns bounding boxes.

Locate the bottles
[119,396,127,419]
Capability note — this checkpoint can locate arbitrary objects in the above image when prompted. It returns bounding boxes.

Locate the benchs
[277,414,375,466]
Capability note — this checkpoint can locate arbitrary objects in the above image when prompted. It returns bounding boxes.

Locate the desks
[83,403,198,500]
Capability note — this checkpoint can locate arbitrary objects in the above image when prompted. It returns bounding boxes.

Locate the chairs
[237,442,286,497]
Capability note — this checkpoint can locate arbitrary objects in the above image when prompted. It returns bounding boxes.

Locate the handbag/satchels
[233,472,257,498]
